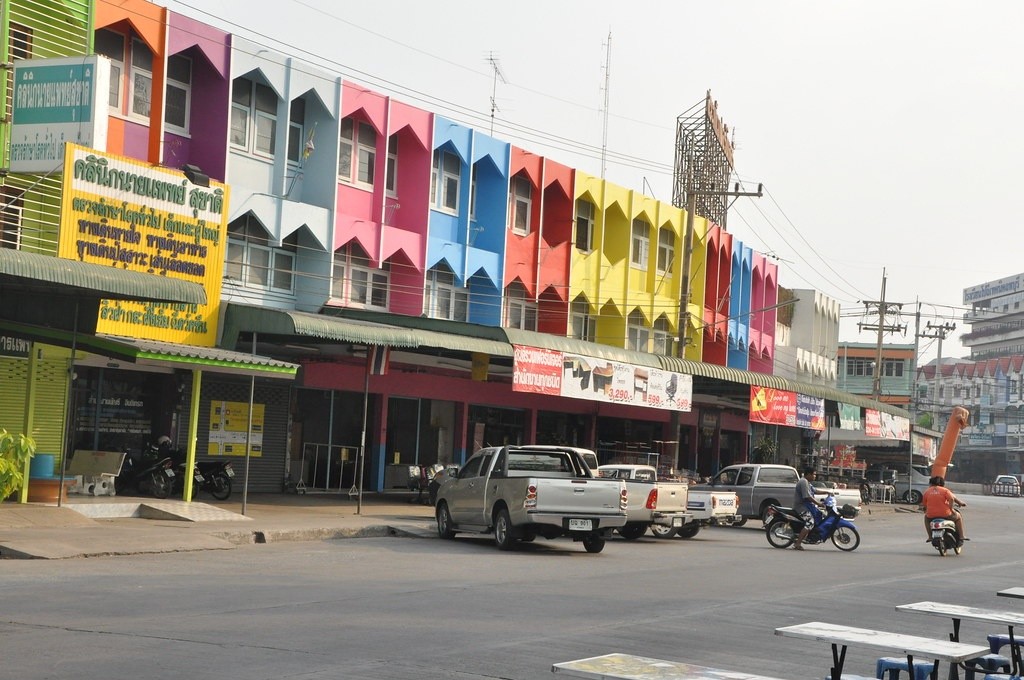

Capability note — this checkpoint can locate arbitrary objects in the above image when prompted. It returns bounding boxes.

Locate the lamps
[163,138,211,189]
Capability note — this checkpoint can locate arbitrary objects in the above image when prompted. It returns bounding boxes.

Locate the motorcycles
[761,492,861,551]
[115,435,233,500]
[930,503,970,557]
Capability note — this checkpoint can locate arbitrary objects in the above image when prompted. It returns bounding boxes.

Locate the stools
[876,657,934,680]
[986,633,1024,654]
[965,655,1011,680]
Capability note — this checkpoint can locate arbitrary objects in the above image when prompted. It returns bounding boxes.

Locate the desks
[894,601,1024,680]
[774,621,991,680]
[551,652,786,680]
[998,586,1024,600]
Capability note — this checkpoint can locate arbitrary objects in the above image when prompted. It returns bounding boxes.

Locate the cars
[992,475,1020,497]
[688,463,800,527]
[893,466,932,505]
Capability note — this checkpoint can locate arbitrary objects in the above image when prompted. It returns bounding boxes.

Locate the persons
[922,476,970,541]
[791,466,831,550]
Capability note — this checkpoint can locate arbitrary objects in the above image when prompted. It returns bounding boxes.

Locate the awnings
[0,246,912,422]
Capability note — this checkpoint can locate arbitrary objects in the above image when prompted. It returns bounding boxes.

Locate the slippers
[960,537,970,540]
[792,544,804,550]
[926,539,933,543]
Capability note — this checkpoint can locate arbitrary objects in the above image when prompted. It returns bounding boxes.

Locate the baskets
[837,504,861,519]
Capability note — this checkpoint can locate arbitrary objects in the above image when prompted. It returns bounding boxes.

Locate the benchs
[61,450,127,497]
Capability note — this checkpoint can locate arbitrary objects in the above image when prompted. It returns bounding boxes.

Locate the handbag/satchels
[145,443,169,460]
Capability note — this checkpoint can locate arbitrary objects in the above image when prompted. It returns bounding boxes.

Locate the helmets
[159,436,172,446]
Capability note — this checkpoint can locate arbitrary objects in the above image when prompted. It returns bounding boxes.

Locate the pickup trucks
[598,463,743,541]
[560,446,694,540]
[434,445,628,553]
